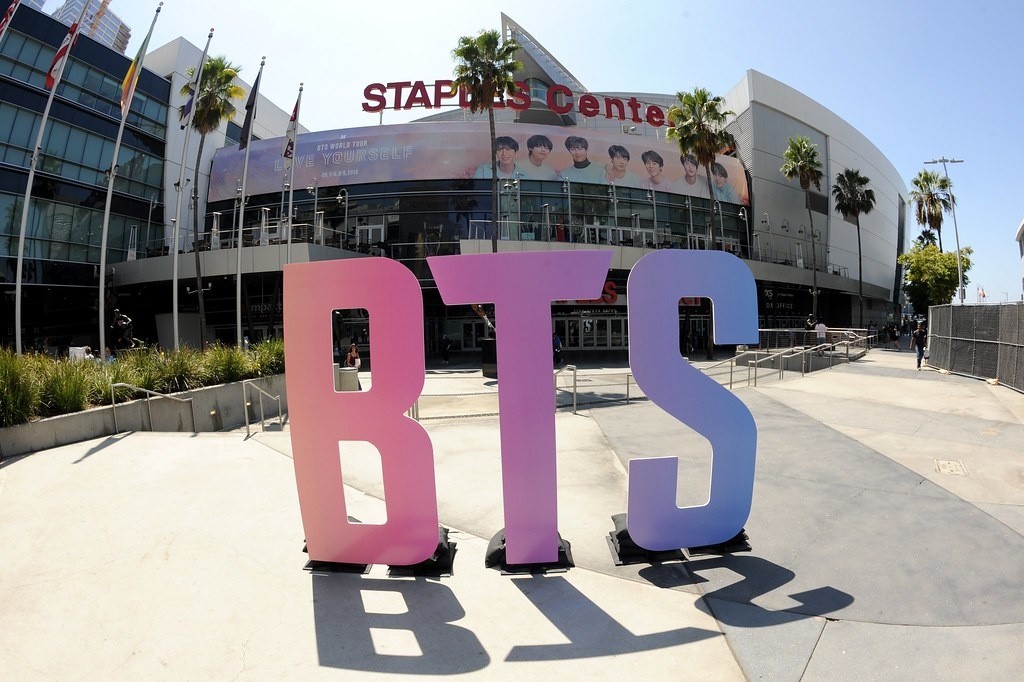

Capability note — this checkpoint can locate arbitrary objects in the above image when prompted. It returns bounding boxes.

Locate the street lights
[684,194,694,250]
[336,189,348,249]
[306,178,319,243]
[923,155,966,306]
[714,201,725,252]
[761,212,773,263]
[798,224,808,269]
[814,228,823,272]
[497,177,511,240]
[645,187,658,249]
[607,182,619,246]
[561,177,572,243]
[186,185,200,253]
[512,171,522,240]
[781,218,792,266]
[232,179,242,249]
[145,193,159,258]
[279,174,290,245]
[739,207,751,260]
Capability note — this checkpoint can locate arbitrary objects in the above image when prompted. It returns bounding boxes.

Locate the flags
[46,0,93,88]
[239,61,265,150]
[0,0,19,36]
[121,6,161,116]
[281,87,303,171]
[977,286,984,297]
[180,33,213,130]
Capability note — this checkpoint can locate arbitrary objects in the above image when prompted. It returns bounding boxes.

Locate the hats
[809,314,814,316]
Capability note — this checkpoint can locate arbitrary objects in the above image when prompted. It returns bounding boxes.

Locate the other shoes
[917,367,921,371]
[885,346,887,348]
[888,347,890,349]
[896,348,900,351]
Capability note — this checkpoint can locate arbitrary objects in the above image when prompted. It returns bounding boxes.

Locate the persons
[473,136,743,204]
[85,346,117,361]
[553,332,563,365]
[806,313,927,371]
[346,343,361,391]
[441,334,450,365]
[110,309,144,347]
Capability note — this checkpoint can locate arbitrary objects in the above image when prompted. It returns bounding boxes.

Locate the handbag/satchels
[924,347,929,359]
[895,330,900,336]
